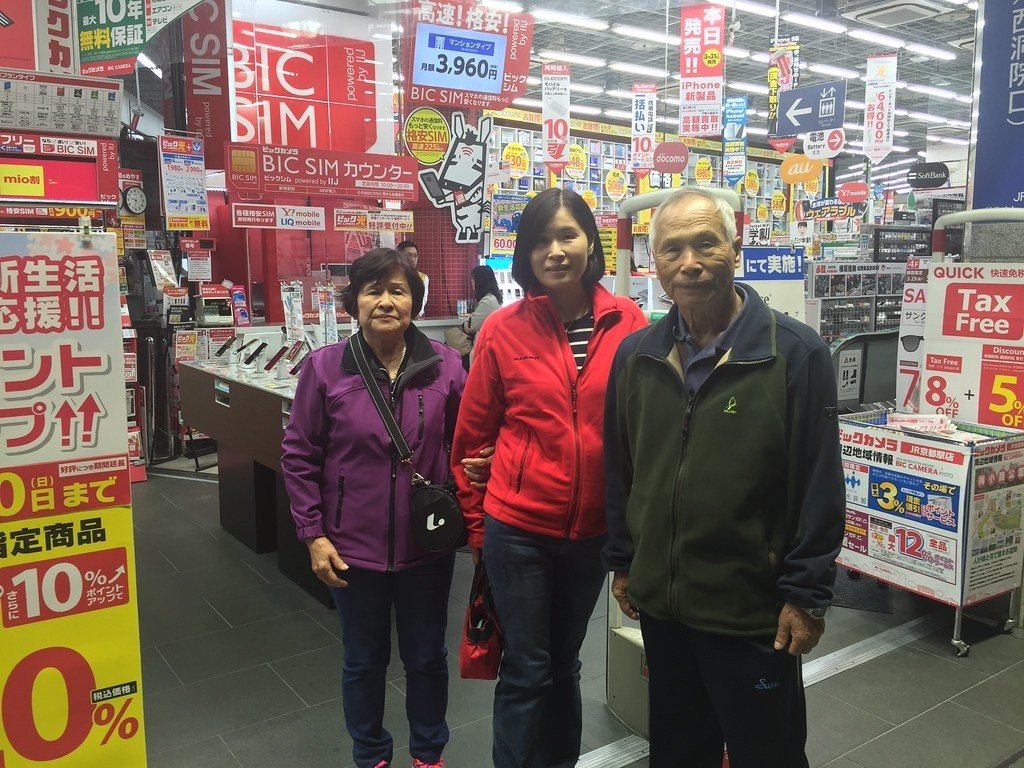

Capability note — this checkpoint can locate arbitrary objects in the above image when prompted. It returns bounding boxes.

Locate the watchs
[801,605,827,620]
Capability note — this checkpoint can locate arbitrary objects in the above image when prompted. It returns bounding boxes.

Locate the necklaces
[564,294,587,332]
[389,341,407,383]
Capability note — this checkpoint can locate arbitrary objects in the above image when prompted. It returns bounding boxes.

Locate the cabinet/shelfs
[482,125,823,258]
[808,262,906,346]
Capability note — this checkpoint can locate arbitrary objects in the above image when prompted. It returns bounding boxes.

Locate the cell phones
[215,336,307,376]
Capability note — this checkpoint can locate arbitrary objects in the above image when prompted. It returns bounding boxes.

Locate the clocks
[123,185,147,214]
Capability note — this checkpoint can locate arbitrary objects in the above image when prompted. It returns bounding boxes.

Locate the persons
[449,186,650,768]
[397,240,503,374]
[281,247,496,768]
[600,191,846,768]
[797,221,808,237]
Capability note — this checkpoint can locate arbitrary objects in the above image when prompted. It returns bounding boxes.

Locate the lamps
[527,6,973,103]
[708,0,956,61]
[513,97,910,153]
[570,82,971,146]
[538,49,972,128]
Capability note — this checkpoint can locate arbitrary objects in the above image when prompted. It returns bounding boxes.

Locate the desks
[194,316,466,344]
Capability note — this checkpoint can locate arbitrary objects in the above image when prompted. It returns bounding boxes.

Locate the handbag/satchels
[409,472,463,553]
[459,559,503,680]
[444,326,473,357]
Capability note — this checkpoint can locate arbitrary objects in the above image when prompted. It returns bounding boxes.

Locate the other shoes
[412,756,444,768]
